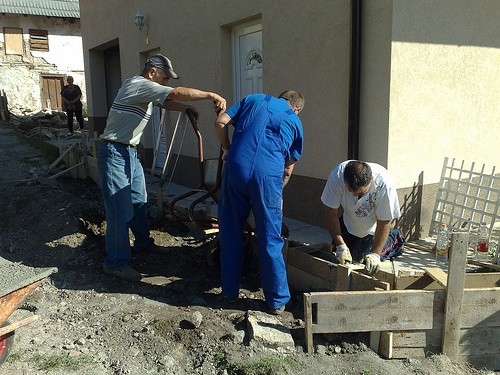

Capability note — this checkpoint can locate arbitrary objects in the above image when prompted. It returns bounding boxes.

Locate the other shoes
[221,292,243,308]
[135,243,169,254]
[271,305,285,314]
[68,132,74,136]
[78,128,84,133]
[103,265,142,281]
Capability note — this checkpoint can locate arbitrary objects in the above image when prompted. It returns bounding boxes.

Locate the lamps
[133,9,150,46]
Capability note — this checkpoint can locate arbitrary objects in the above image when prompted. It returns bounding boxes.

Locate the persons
[60,76,85,136]
[320,160,401,277]
[95,53,226,281]
[214,89,305,314]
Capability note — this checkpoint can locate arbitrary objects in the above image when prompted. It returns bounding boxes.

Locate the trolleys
[169,101,290,251]
[0,262,59,366]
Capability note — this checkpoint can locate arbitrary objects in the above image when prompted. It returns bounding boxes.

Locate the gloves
[336,244,352,264]
[364,253,380,275]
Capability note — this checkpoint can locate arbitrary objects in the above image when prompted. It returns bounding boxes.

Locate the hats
[145,55,180,79]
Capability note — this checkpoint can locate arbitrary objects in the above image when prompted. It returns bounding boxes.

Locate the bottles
[436,223,449,258]
[477,222,490,262]
